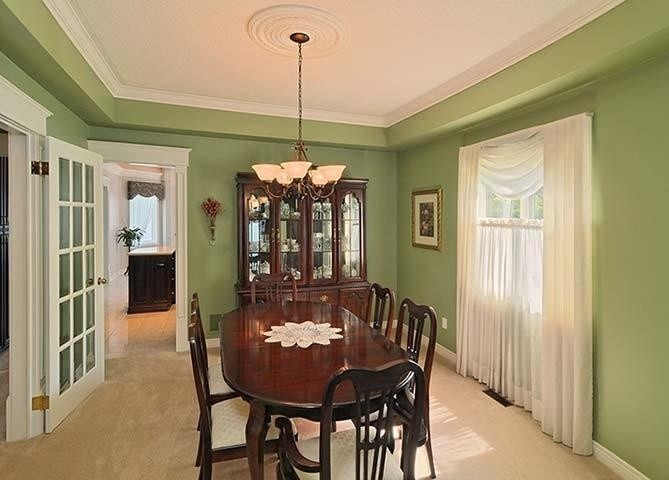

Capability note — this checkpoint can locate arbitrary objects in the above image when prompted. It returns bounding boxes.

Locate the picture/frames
[411,187,442,252]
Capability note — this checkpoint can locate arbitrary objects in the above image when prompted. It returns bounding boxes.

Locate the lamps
[251,27,346,213]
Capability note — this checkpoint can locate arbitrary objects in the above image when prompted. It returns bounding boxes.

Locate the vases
[209,223,215,245]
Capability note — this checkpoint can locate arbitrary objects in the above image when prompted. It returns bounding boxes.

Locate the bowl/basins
[281,238,300,253]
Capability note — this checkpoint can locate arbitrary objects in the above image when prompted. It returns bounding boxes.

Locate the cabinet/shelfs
[234,171,373,326]
[128,251,176,314]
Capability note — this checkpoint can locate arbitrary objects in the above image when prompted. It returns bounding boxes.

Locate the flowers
[201,194,225,225]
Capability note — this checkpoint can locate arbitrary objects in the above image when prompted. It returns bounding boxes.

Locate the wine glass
[312,202,330,220]
